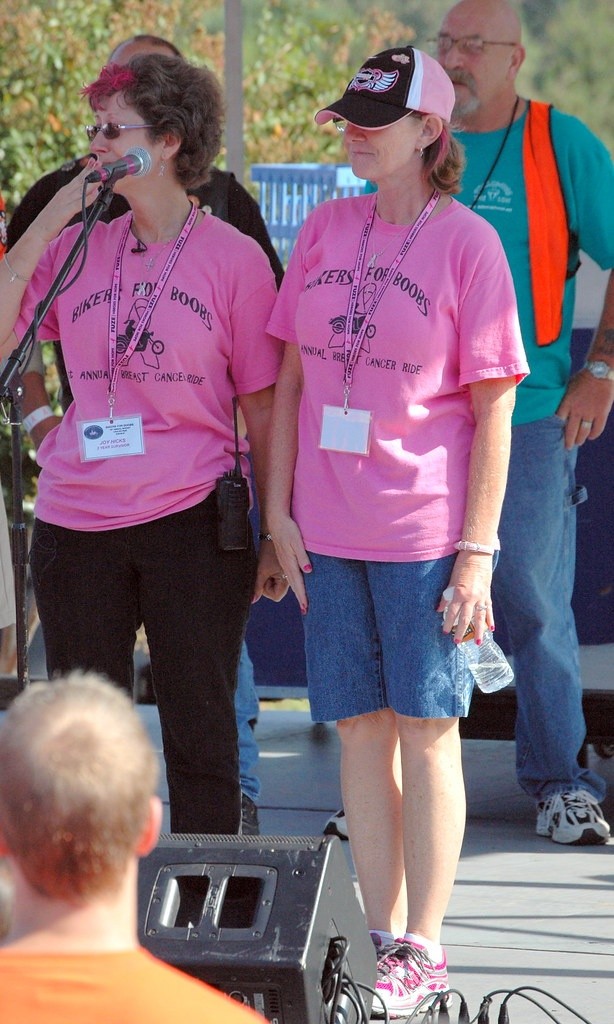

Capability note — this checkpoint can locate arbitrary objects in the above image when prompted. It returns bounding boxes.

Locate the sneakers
[323,809,348,840]
[536,790,611,845]
[368,933,453,1020]
[241,795,260,835]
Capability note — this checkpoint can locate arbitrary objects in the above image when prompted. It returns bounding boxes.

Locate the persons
[0,666,271,1024]
[0,54,276,933]
[262,44,529,1016]
[0,29,286,837]
[359,0,614,844]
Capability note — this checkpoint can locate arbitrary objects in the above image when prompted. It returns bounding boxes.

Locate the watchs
[583,359,613,381]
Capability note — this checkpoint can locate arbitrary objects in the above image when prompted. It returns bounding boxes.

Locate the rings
[474,605,487,610]
[281,573,288,578]
[581,421,592,428]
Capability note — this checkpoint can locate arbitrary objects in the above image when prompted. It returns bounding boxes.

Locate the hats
[314,46,455,131]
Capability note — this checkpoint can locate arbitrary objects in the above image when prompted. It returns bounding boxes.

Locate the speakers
[136,833,377,1024]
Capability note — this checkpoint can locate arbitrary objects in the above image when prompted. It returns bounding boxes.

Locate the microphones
[84,145,152,183]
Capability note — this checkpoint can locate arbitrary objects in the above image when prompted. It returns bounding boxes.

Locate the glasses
[333,117,348,132]
[86,123,165,143]
[427,34,517,55]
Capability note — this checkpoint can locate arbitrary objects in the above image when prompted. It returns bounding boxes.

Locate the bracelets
[23,404,55,434]
[256,532,272,541]
[453,541,494,555]
[3,253,32,283]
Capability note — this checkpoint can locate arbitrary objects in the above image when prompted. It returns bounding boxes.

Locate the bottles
[442,585,514,694]
